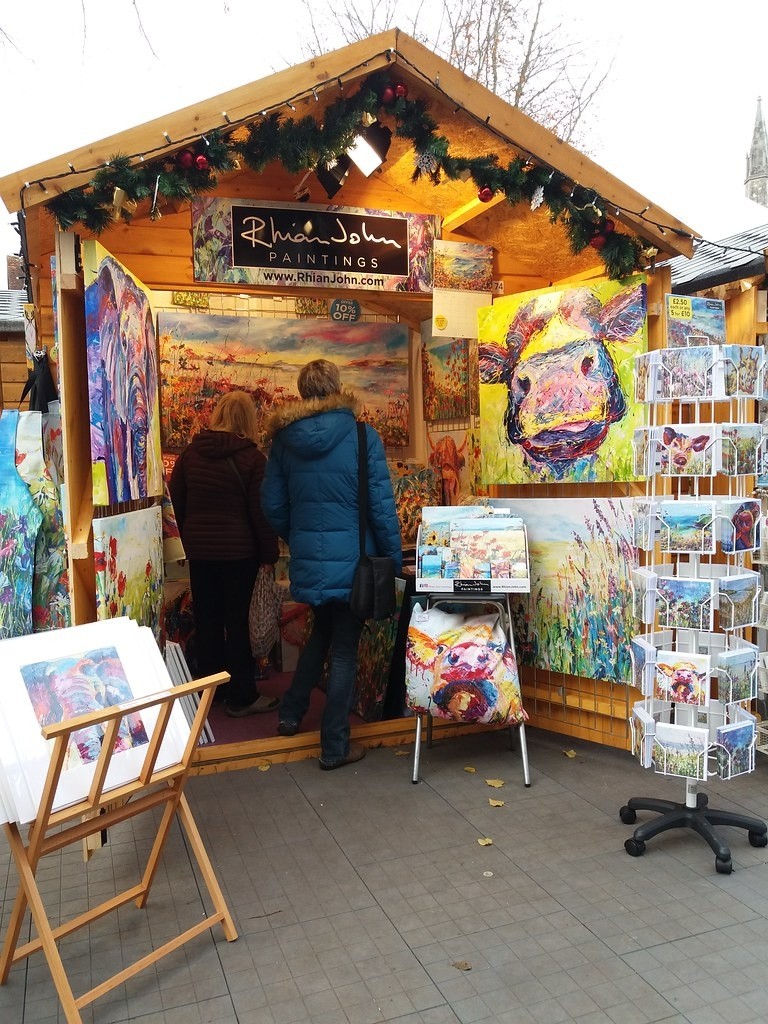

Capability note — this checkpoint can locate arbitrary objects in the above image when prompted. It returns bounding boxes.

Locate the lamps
[344,126,392,178]
[316,154,354,200]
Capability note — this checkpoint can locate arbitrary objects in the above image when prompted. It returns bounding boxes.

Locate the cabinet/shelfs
[618,334,768,875]
[412,525,531,788]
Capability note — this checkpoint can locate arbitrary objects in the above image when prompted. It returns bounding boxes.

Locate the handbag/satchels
[348,556,397,621]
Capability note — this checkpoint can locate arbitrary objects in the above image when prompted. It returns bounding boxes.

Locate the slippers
[226,696,281,717]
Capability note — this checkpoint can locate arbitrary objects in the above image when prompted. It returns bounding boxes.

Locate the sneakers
[278,718,302,736]
[320,741,366,769]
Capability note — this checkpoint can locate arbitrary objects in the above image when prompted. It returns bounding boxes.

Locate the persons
[169,390,282,719]
[260,359,403,768]
